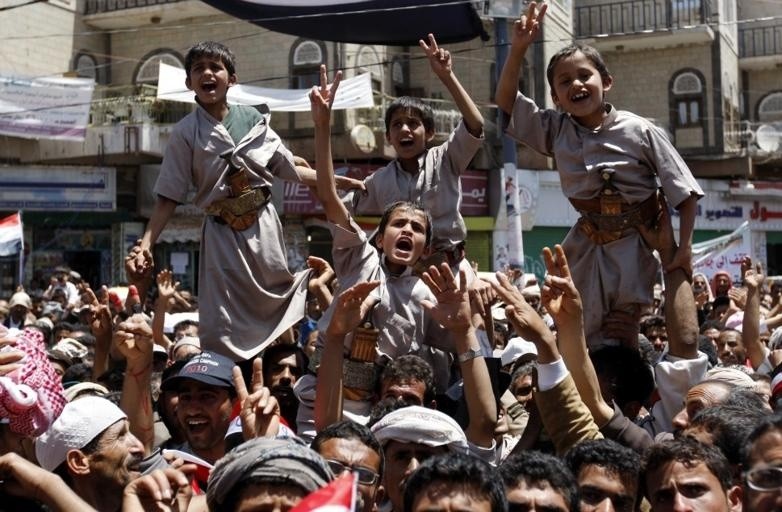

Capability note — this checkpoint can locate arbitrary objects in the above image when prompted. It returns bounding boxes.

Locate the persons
[590,192,706,440]
[494,1,703,351]
[293,31,496,365]
[293,63,437,446]
[0,241,782,512]
[123,40,367,390]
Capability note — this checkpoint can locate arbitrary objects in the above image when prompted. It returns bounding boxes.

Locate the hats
[33,395,129,474]
[158,347,245,395]
[8,291,33,310]
[370,404,470,457]
[205,435,338,512]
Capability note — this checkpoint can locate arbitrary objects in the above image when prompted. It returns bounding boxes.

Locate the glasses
[324,458,381,485]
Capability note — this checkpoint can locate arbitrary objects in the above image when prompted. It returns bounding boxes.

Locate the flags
[0,209,25,256]
